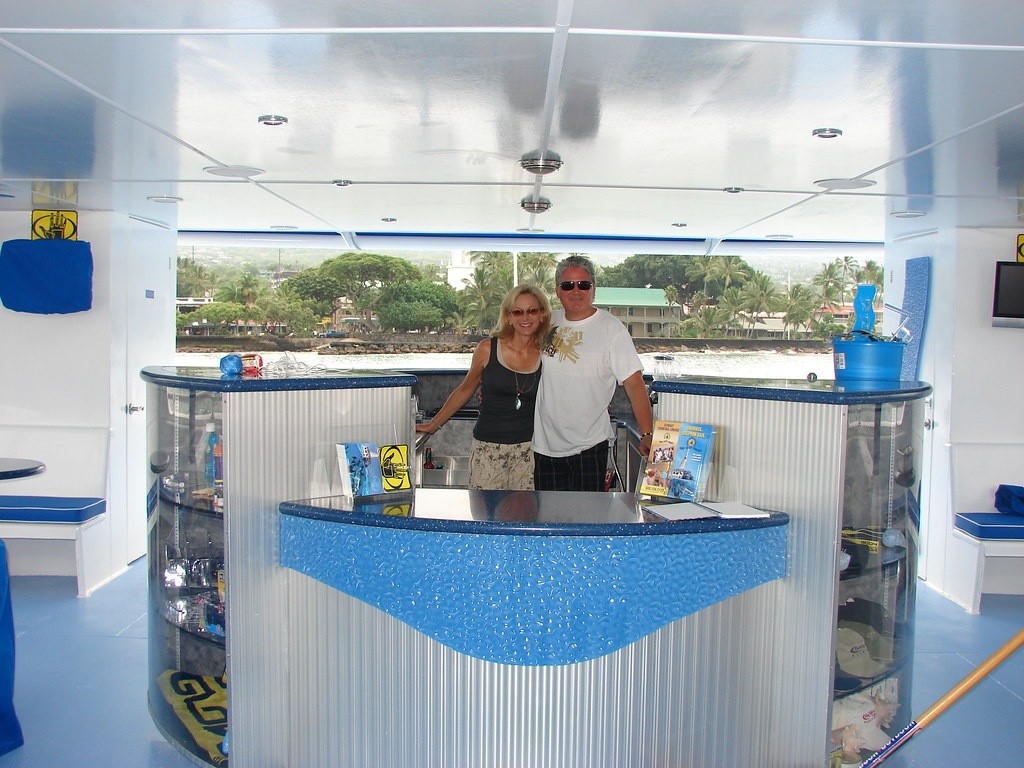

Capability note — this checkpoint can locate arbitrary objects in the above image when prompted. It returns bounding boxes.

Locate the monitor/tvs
[992,261,1024,319]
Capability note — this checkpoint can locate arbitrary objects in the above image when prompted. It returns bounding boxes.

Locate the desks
[0,458,46,482]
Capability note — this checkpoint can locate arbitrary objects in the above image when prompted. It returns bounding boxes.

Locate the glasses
[514,372,522,410]
[558,281,594,290]
[511,308,544,316]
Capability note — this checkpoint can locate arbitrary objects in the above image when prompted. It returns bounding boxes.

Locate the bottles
[203,423,219,481]
[424,447,434,469]
[214,492,217,504]
[214,434,223,480]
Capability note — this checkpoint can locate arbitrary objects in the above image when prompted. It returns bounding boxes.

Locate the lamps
[520,159,564,175]
[522,202,550,214]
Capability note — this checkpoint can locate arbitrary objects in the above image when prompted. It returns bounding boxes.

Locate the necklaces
[509,335,537,410]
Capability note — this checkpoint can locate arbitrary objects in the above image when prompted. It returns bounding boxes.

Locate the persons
[473,255,654,492]
[652,447,674,463]
[415,284,553,491]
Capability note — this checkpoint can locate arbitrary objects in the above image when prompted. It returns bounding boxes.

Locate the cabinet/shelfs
[138,363,229,768]
[824,379,932,767]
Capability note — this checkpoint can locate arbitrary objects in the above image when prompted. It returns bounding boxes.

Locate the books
[643,500,771,523]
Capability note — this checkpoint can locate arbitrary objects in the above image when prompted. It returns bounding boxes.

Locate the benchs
[944,439,1024,614]
[1,423,114,599]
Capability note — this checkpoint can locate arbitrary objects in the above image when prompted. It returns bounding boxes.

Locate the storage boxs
[832,340,908,382]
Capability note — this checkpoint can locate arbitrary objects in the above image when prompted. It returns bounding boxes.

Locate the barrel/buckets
[832,340,906,380]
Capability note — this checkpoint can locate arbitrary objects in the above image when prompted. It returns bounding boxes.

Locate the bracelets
[431,417,442,430]
[640,432,652,440]
